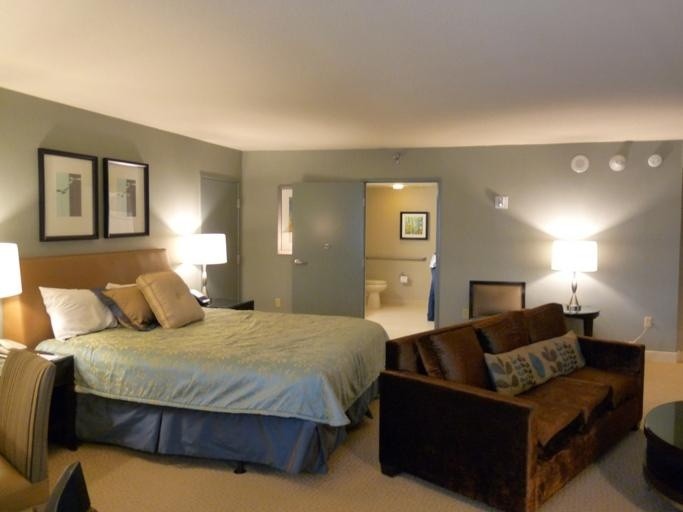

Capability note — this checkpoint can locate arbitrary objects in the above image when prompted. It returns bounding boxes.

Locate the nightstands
[0,343,76,452]
[205,298,254,310]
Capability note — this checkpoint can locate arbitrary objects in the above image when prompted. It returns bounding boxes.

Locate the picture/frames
[37,147,99,241]
[103,156,149,237]
[399,212,428,240]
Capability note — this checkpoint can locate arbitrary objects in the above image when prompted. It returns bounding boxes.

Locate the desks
[564,309,600,336]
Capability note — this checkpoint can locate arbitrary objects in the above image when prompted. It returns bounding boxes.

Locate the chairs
[469,281,525,319]
[0,348,63,512]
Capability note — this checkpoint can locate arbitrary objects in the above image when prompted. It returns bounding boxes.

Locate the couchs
[380,303,646,512]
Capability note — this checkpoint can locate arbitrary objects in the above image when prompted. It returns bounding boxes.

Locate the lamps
[188,233,227,300]
[550,240,598,312]
[0,242,23,299]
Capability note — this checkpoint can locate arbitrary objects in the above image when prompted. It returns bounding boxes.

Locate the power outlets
[644,317,653,328]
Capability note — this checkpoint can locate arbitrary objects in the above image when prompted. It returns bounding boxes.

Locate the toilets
[365,279,387,310]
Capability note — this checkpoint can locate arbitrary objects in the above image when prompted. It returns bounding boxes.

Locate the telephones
[0,338,28,354]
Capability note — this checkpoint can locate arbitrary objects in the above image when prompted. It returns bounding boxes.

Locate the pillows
[90,284,159,332]
[134,270,205,329]
[38,286,120,342]
[416,303,584,397]
[104,280,136,291]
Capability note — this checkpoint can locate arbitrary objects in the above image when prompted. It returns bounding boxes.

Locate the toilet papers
[400,276,408,283]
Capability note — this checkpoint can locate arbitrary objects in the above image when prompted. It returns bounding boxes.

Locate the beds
[2,247,390,474]
